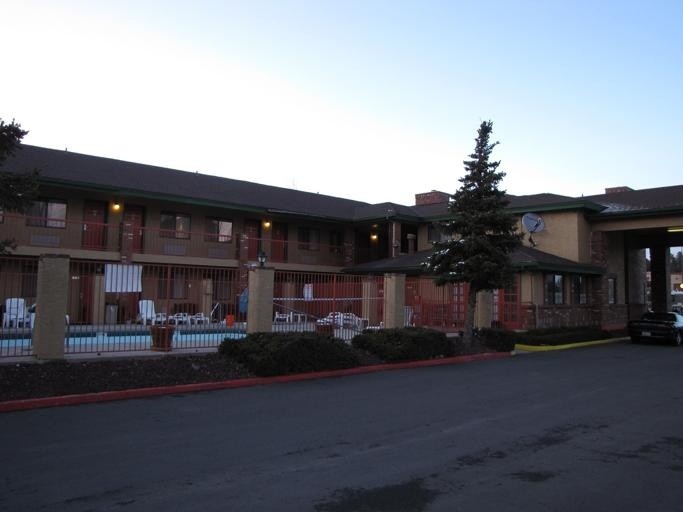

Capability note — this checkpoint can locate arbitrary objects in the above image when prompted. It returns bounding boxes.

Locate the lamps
[258,251,268,267]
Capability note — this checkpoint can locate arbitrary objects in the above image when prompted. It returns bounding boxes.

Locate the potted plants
[150,324,175,351]
[315,323,335,338]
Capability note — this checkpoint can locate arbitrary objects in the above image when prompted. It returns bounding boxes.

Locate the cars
[628,308,683,345]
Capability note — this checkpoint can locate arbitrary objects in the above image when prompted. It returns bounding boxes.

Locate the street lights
[256,251,267,268]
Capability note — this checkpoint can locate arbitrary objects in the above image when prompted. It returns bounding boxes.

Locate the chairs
[139,299,156,326]
[6,298,27,328]
